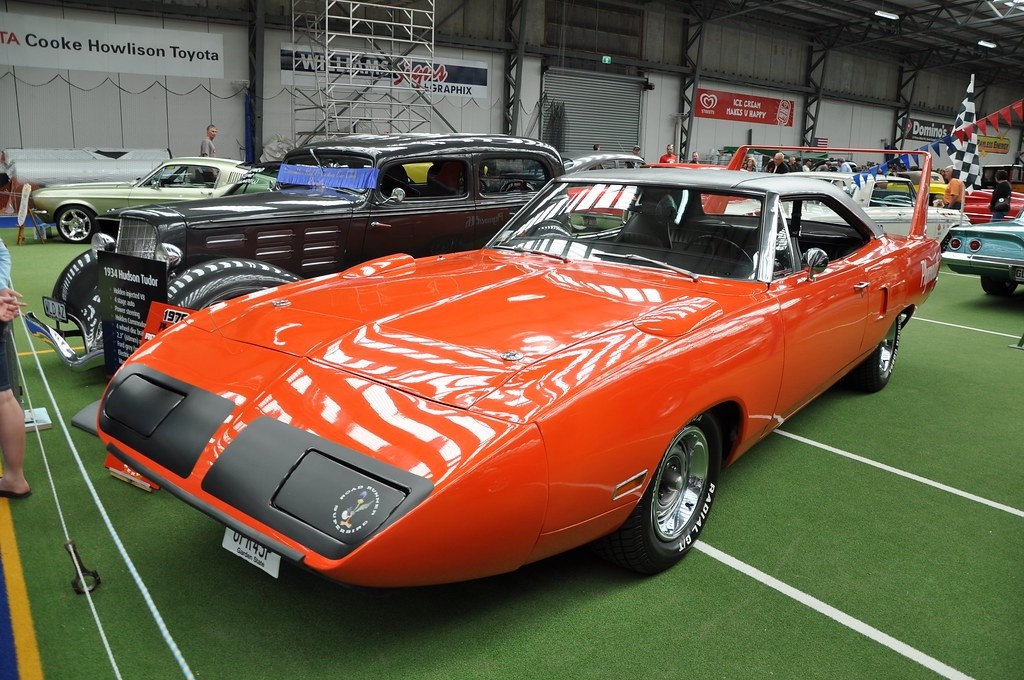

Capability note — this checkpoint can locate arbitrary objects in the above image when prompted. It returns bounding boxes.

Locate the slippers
[0,476,31,499]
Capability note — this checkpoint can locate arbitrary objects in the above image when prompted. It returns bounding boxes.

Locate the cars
[941,207,1024,294]
[880,165,1024,223]
[31,157,249,244]
[97,144,941,588]
[26,133,566,372]
[722,172,972,244]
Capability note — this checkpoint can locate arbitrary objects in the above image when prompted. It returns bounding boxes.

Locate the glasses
[634,149,640,151]
[773,158,782,161]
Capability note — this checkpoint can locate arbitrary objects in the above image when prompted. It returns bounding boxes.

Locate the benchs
[669,222,856,262]
[479,174,545,193]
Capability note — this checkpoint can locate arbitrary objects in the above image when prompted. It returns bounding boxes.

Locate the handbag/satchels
[989,195,1010,212]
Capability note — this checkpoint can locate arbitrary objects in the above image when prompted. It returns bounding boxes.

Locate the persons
[741,152,948,184]
[943,165,964,210]
[659,143,677,164]
[589,145,600,169]
[201,124,218,157]
[1,170,36,498]
[689,152,703,164]
[625,145,644,169]
[987,170,1013,223]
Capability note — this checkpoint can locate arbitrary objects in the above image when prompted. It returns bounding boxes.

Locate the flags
[947,79,982,196]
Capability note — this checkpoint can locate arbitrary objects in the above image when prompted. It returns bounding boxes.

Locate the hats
[748,157,757,165]
[633,145,640,149]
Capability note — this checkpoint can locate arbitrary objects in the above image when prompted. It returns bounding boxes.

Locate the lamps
[874,0,899,20]
[977,29,996,48]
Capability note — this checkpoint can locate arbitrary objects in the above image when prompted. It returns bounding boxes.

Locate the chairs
[741,224,792,271]
[420,165,463,196]
[202,172,216,182]
[612,191,677,249]
[381,164,410,197]
